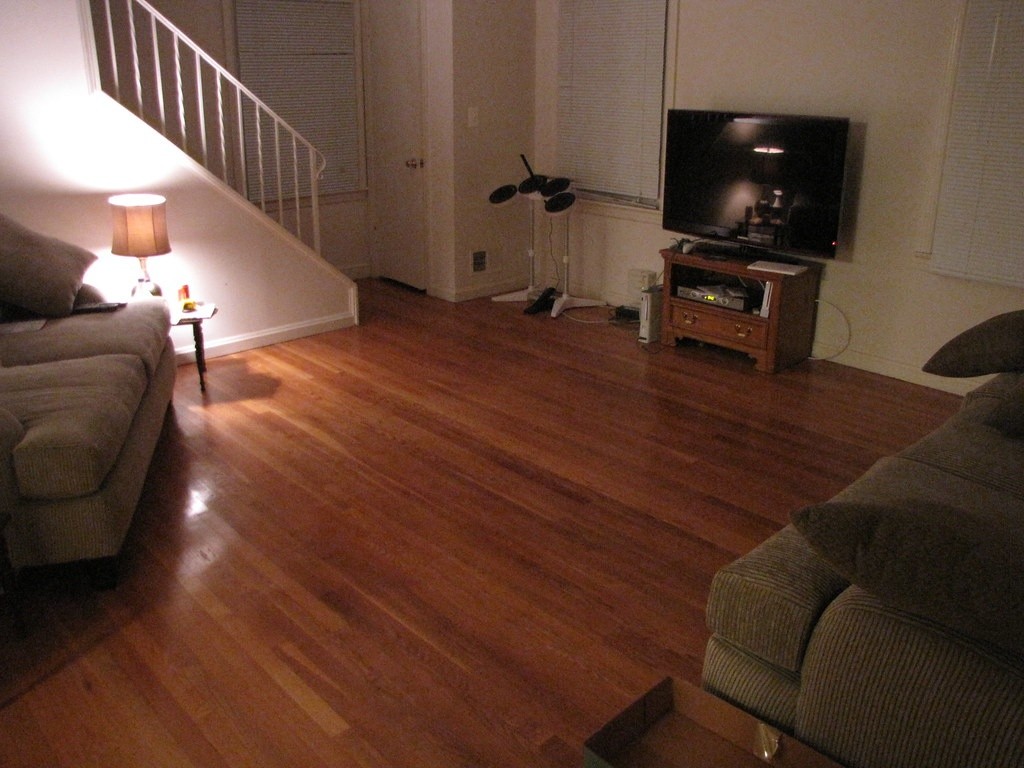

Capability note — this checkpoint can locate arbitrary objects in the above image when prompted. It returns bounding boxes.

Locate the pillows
[791,498,1024,658]
[922,309,1024,378]
[0,210,98,317]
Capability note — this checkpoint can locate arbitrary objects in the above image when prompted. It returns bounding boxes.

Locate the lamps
[107,193,171,297]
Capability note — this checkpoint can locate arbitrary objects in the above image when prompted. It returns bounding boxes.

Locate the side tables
[178,308,217,391]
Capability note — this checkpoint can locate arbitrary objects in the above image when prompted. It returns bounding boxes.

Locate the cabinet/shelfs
[659,247,824,376]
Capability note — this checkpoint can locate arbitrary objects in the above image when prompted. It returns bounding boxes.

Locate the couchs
[0,285,177,590]
[701,373,1024,768]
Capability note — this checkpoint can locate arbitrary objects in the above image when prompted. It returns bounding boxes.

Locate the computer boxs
[637,283,664,344]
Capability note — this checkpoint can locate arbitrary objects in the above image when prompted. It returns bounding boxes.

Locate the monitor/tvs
[662,109,850,265]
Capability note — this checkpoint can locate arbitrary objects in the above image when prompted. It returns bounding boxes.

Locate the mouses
[683,242,695,254]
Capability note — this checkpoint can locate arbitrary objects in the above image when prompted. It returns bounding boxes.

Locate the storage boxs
[585,674,845,767]
